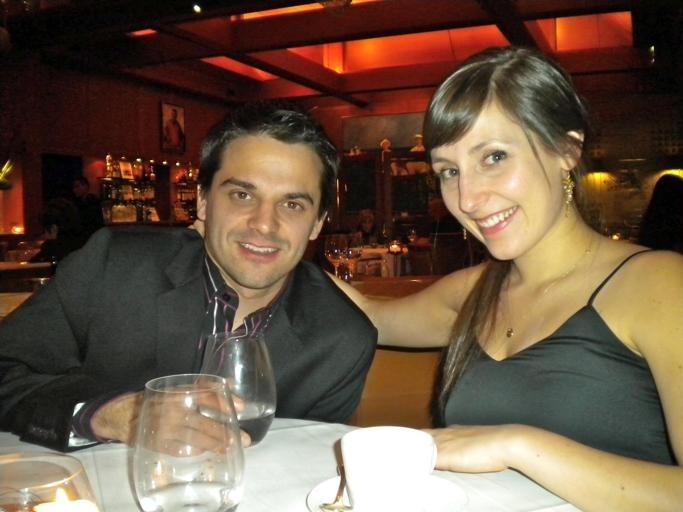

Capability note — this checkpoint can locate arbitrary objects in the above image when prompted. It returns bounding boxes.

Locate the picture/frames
[158,103,187,155]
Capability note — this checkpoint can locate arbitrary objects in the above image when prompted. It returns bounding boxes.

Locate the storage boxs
[170,163,199,226]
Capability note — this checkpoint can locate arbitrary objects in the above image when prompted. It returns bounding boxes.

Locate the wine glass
[133,373,244,512]
[199,330,277,448]
[347,233,364,281]
[0,451,103,512]
[328,233,348,281]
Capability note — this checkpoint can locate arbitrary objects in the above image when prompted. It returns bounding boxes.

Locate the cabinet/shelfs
[99,155,165,221]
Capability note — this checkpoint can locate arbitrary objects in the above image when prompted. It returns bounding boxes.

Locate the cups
[340,426,437,509]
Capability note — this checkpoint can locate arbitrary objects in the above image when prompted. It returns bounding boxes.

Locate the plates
[306,475,469,511]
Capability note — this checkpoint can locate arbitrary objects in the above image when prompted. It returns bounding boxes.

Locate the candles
[389,236,404,279]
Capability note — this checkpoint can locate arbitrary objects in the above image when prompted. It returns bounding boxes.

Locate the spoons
[319,466,350,512]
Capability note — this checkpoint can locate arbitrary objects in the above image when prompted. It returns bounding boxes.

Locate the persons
[70,176,105,232]
[349,210,384,246]
[163,108,185,150]
[1,106,378,460]
[29,215,68,263]
[327,45,683,512]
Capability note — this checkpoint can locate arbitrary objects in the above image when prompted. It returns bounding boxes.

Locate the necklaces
[503,235,594,338]
